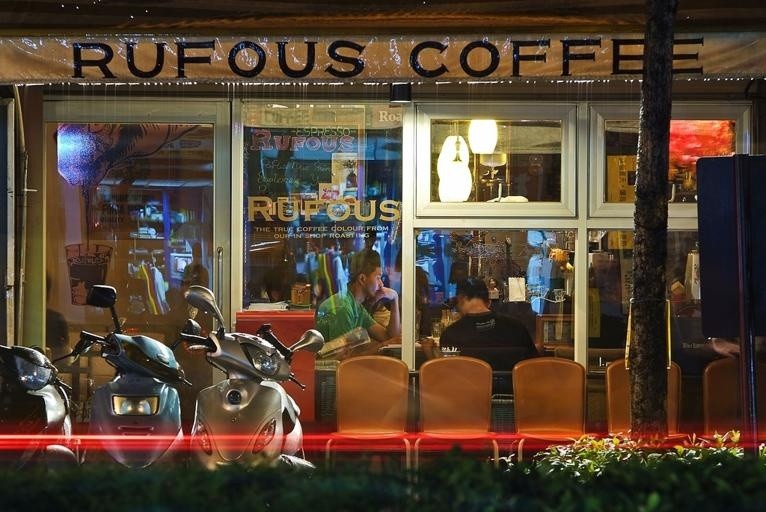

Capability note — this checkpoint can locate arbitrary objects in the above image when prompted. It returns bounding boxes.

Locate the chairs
[509,355,593,462]
[414,355,500,473]
[322,354,412,472]
[603,357,685,441]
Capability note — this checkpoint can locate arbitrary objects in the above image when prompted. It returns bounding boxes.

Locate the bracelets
[706,335,712,344]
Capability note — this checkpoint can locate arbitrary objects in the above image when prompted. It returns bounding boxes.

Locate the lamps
[436,120,498,202]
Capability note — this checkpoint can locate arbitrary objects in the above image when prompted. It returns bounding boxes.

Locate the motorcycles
[179,285,324,471]
[1,284,202,473]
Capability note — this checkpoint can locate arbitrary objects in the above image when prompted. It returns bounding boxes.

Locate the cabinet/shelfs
[78,175,213,316]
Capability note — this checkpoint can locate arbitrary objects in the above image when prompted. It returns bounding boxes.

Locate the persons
[640,296,742,426]
[182,243,209,320]
[487,278,499,307]
[316,248,400,356]
[421,274,543,398]
